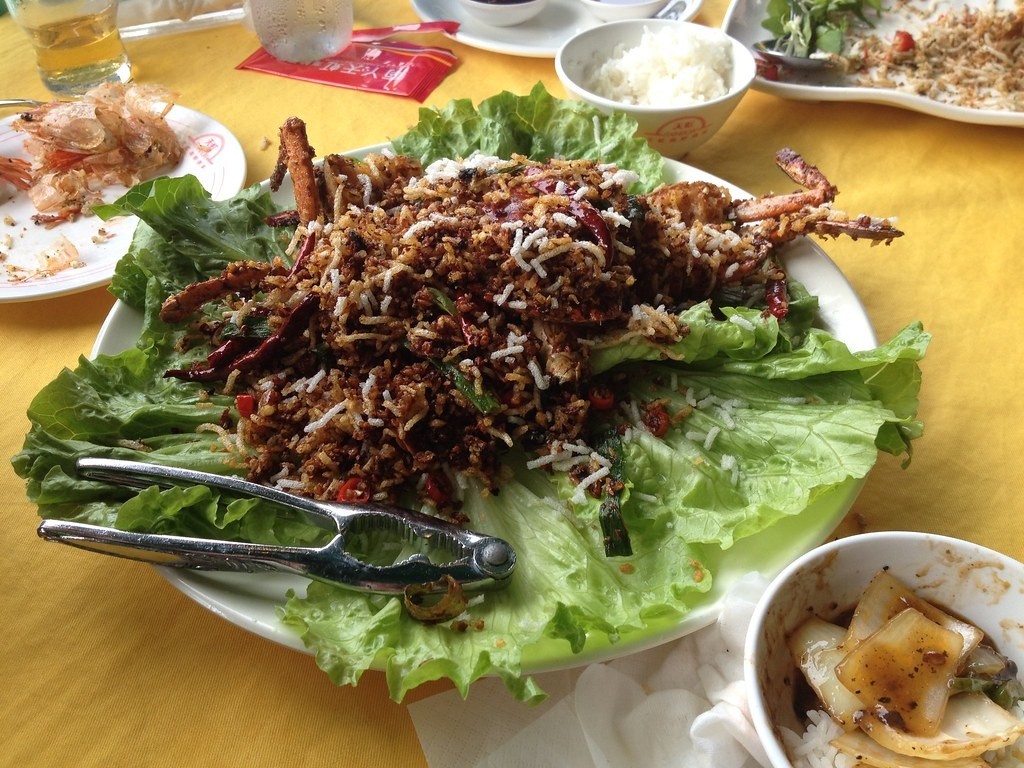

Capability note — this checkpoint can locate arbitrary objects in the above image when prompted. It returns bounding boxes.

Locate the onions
[788,572,1024,768]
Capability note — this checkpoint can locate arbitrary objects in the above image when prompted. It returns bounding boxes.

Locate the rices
[573,22,738,107]
[778,708,1024,768]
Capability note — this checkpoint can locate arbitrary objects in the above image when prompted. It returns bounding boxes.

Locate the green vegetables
[758,0,887,61]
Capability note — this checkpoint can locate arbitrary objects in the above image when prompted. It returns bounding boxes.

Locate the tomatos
[893,30,915,52]
[755,59,777,82]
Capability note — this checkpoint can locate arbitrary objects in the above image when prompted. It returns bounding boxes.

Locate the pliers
[35,457,518,599]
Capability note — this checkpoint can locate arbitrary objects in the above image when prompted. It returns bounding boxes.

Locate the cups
[3,0,136,98]
[247,0,354,64]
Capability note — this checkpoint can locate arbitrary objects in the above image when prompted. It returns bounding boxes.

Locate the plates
[720,0,1024,128]
[87,138,885,678]
[411,0,704,59]
[0,99,247,303]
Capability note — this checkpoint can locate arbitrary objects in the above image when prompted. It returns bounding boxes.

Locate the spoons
[750,0,831,70]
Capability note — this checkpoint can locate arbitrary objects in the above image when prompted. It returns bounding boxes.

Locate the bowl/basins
[743,530,1024,768]
[554,18,757,163]
[458,0,547,28]
[580,0,671,24]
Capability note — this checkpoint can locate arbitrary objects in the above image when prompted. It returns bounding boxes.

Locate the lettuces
[9,83,934,707]
